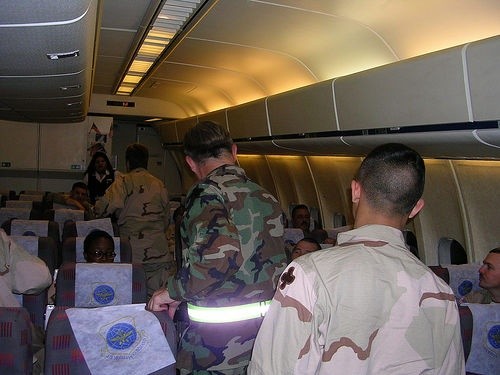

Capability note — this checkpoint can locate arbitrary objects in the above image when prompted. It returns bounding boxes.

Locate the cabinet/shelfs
[0,120,88,179]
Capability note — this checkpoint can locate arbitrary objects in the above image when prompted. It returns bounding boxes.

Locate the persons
[246,143,468,374]
[461,248,500,303]
[283,204,338,264]
[0,152,117,307]
[146,121,290,375]
[96,145,171,298]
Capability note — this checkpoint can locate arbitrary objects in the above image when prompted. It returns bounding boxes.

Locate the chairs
[0,191,500,375]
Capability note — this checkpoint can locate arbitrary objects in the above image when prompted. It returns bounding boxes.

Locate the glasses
[89,251,116,259]
